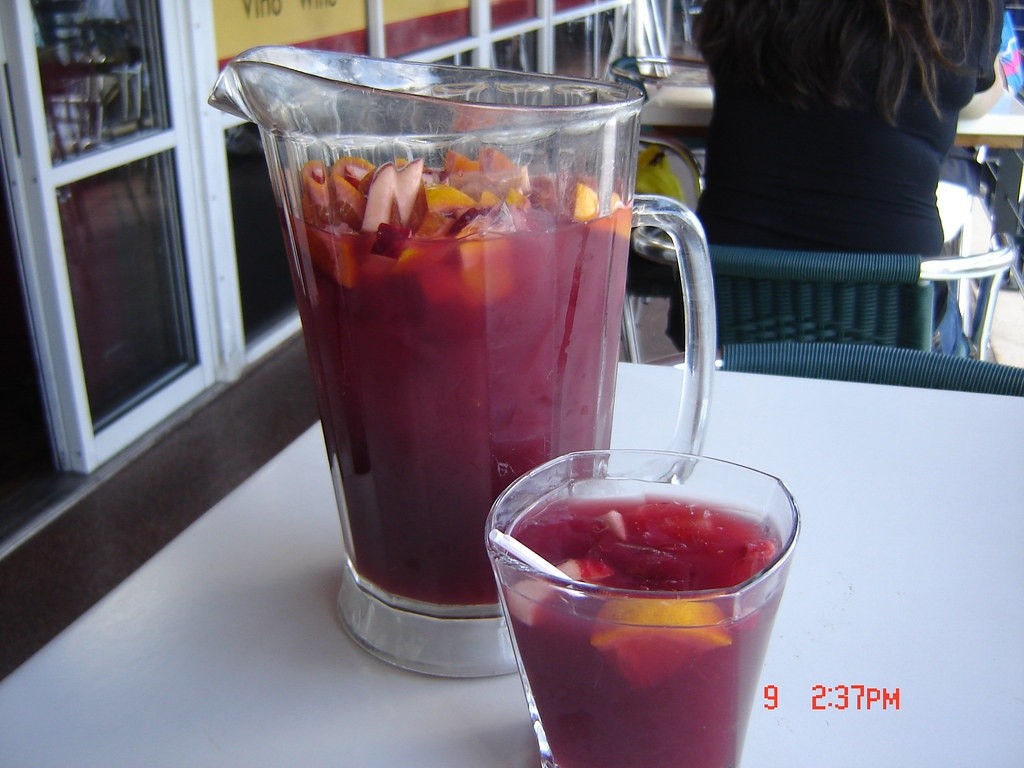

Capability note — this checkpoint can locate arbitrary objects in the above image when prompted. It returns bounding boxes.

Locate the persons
[663,0,1007,359]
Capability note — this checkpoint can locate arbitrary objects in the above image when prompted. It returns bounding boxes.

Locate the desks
[639,85,1024,152]
[0,362,1024,768]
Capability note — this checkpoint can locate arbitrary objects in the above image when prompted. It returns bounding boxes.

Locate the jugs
[204,44,720,680]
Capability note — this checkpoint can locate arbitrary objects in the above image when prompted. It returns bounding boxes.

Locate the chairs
[673,342,1024,397]
[610,55,709,101]
[620,228,1016,364]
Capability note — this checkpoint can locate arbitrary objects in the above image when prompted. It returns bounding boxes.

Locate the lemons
[589,596,735,688]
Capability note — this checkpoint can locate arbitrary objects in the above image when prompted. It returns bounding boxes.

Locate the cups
[484,448,801,768]
